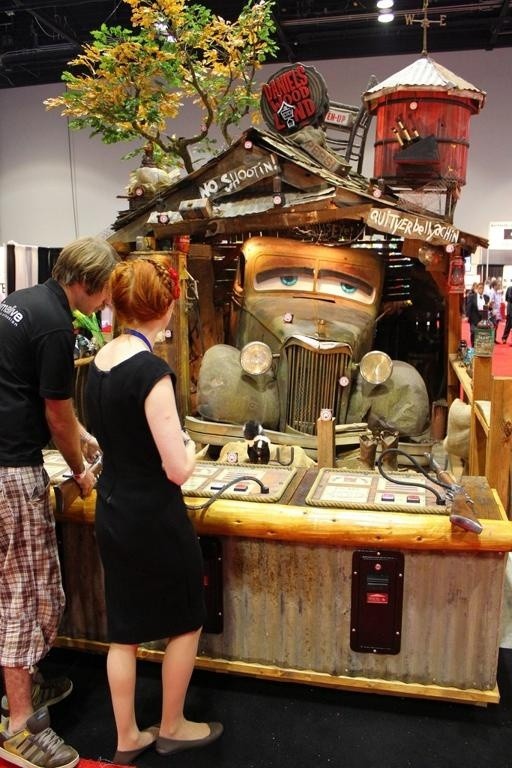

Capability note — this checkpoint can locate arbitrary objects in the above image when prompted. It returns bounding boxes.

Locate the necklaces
[119,327,153,354]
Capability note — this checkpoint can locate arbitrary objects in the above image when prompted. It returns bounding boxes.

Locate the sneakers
[2,672,73,710]
[0,705,80,768]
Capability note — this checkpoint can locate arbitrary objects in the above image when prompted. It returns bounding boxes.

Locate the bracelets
[72,470,86,480]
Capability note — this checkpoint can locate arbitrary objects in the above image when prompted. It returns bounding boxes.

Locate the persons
[466,277,512,348]
[0,236,123,768]
[85,255,225,764]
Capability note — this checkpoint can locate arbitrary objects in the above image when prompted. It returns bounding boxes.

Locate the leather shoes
[113,723,161,765]
[156,722,224,756]
